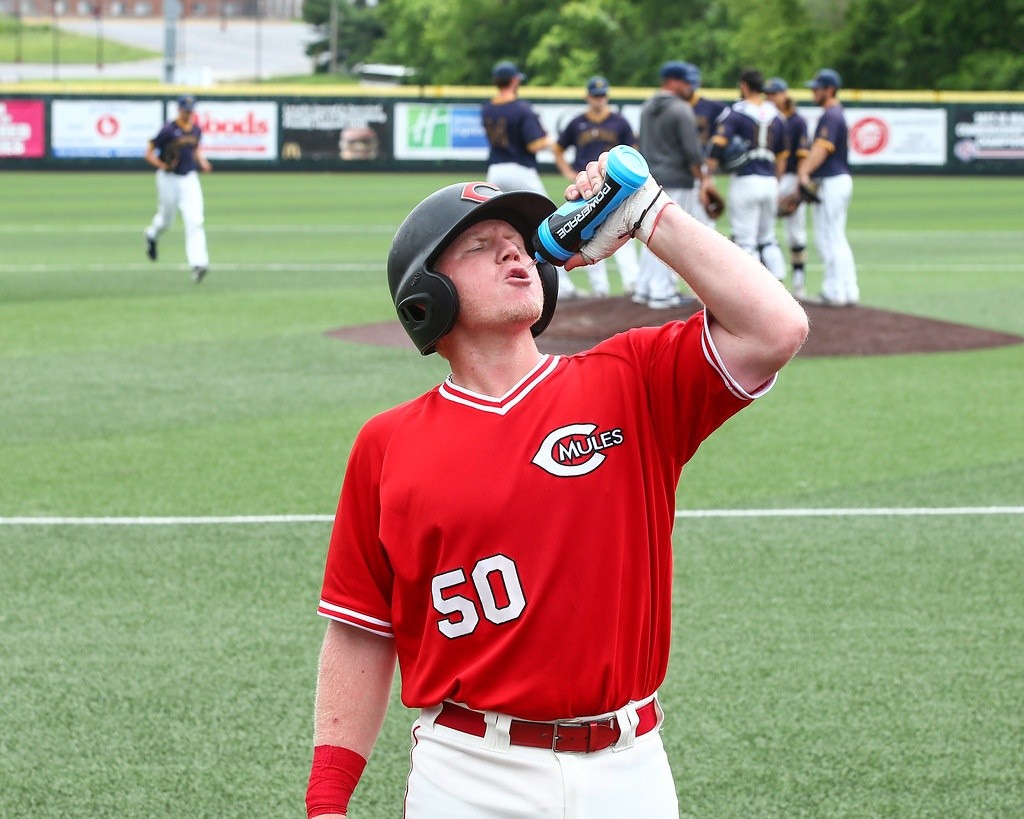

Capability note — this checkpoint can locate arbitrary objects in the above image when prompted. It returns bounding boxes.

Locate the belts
[434,701,657,753]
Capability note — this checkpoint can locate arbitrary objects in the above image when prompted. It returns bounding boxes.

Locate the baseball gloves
[704,189,723,218]
[799,184,820,203]
[164,143,180,171]
[779,195,799,216]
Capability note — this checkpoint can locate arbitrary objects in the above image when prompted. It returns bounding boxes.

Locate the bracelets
[618,185,663,239]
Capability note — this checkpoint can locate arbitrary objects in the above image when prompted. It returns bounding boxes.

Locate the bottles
[531,144,650,267]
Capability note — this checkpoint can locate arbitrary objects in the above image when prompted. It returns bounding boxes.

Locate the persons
[144,95,210,282]
[305,146,810,819]
[480,59,862,309]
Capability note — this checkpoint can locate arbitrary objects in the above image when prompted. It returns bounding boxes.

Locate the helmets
[386,181,560,356]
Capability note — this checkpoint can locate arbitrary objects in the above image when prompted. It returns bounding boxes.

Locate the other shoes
[193,266,207,284]
[145,230,158,261]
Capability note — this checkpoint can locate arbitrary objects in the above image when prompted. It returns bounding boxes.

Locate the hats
[493,61,526,80]
[805,69,841,89]
[762,78,788,94]
[661,61,700,88]
[587,75,610,96]
[178,95,196,112]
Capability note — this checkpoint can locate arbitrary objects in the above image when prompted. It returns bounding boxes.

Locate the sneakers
[632,292,690,309]
[803,294,854,309]
